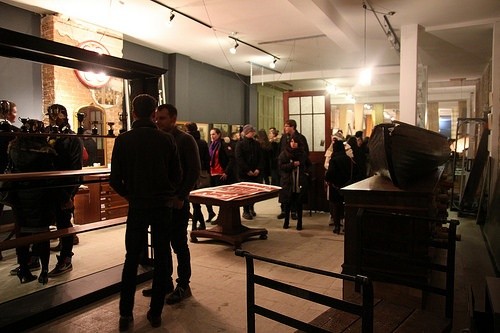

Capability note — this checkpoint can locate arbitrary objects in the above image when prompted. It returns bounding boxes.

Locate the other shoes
[143,282,175,296]
[166,285,191,303]
[51,242,63,251]
[251,209,256,216]
[243,212,252,220]
[49,261,72,276]
[146,311,161,327]
[196,223,207,229]
[296,224,302,230]
[206,212,215,222]
[277,213,286,219]
[118,316,133,330]
[329,223,335,226]
[291,213,296,220]
[10,261,39,274]
[283,224,289,228]
[72,236,79,245]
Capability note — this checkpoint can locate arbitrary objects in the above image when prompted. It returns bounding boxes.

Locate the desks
[340,166,462,308]
[188,181,283,250]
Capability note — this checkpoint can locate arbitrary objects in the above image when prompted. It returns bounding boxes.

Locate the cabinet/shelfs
[0,28,168,333]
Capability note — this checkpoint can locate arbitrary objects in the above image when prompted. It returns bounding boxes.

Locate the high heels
[37,270,48,285]
[334,227,340,234]
[15,269,38,282]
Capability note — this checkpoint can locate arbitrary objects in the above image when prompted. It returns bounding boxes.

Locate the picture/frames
[74,40,113,89]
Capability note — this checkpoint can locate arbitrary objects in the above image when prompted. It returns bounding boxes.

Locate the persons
[0,100,82,285]
[82,130,98,165]
[277,120,310,220]
[277,135,312,230]
[176,120,281,232]
[141,103,202,305]
[108,95,182,333]
[323,129,370,235]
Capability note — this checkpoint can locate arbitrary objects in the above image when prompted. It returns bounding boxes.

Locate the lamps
[362,2,396,15]
[270,56,277,68]
[324,82,355,102]
[230,39,239,54]
[165,10,175,27]
[386,27,399,50]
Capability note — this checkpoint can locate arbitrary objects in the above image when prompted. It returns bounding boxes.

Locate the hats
[45,103,66,115]
[242,124,255,135]
